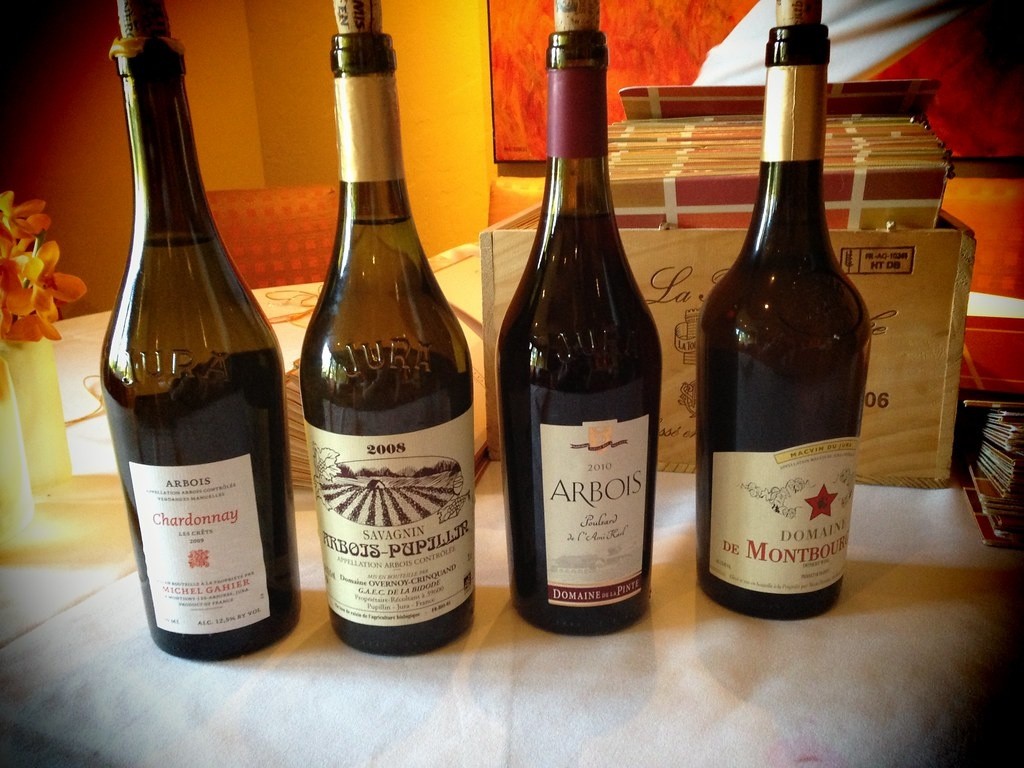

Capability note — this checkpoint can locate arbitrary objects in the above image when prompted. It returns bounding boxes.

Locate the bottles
[99,0,870,661]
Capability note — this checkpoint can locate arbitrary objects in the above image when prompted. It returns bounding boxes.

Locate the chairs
[207,186,339,290]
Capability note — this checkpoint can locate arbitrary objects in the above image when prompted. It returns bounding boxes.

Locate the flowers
[0,191,88,343]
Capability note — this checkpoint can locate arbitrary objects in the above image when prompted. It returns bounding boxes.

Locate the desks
[0,244,1024,768]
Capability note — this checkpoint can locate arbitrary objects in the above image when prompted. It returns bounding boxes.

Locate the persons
[684,0,1024,299]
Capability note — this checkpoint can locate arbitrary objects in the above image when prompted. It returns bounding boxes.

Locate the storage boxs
[478,202,977,488]
